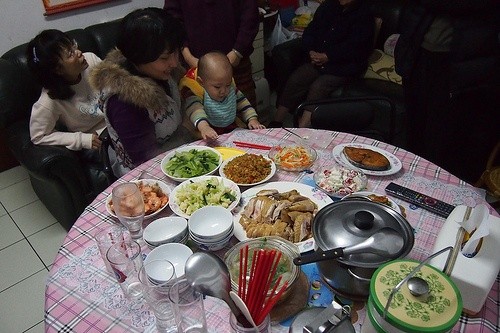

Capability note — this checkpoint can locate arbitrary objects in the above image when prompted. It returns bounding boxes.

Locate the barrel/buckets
[359,246,463,333]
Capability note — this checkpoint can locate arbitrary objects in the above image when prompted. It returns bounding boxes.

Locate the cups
[105,241,144,303]
[138,259,178,333]
[94,224,130,274]
[110,183,145,244]
[168,278,208,333]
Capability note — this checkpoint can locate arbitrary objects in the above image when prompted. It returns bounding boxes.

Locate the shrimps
[108,181,169,217]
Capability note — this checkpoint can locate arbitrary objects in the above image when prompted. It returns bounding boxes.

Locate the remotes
[385,182,455,219]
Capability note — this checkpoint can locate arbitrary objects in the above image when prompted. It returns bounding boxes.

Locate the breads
[343,146,390,170]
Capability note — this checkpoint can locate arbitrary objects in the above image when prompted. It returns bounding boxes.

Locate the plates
[232,181,335,254]
[160,146,222,182]
[268,144,317,171]
[333,142,403,176]
[106,179,172,220]
[169,176,241,219]
[220,152,276,186]
[314,162,368,198]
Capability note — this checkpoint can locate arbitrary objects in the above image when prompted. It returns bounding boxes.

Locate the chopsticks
[236,244,290,326]
[232,139,272,150]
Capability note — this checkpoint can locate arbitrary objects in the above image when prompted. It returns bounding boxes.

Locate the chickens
[239,190,319,243]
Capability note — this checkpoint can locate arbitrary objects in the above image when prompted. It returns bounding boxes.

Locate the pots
[310,200,416,299]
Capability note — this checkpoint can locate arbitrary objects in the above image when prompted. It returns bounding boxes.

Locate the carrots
[275,147,311,169]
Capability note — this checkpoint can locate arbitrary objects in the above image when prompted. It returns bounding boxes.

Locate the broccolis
[175,178,237,216]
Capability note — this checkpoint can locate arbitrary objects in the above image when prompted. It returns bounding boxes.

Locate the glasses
[65,39,78,61]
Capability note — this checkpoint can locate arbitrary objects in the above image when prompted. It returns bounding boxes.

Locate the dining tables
[45,128,500,333]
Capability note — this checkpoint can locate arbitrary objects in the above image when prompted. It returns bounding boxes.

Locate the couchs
[270,0,500,186]
[0,19,118,231]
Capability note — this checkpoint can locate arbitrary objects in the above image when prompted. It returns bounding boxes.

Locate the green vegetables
[165,148,219,179]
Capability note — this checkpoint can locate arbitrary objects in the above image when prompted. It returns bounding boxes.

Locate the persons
[28,30,117,163]
[261,0,500,211]
[179,51,266,140]
[164,0,259,126]
[93,7,187,177]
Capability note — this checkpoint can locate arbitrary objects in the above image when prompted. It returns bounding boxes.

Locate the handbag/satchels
[270,14,297,50]
[367,49,402,84]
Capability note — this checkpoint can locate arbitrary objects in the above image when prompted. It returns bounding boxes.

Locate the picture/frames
[41,0,112,16]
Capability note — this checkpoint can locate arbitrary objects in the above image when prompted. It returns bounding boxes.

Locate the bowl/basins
[144,243,193,295]
[221,235,300,305]
[143,216,189,251]
[188,205,235,252]
